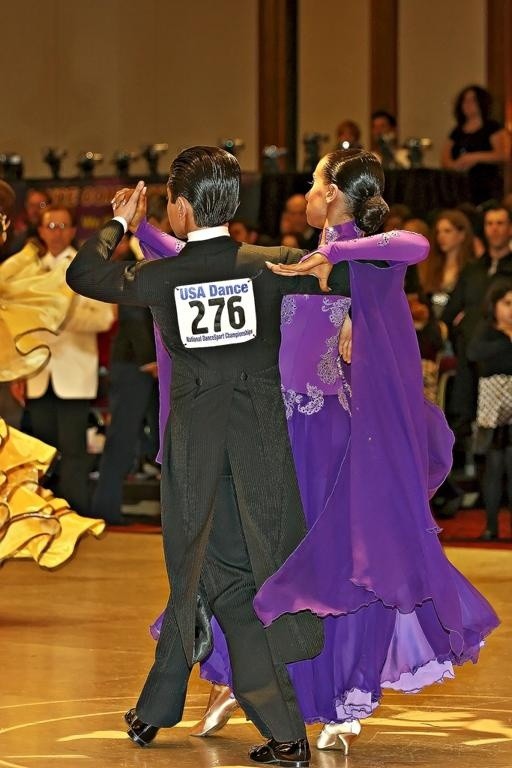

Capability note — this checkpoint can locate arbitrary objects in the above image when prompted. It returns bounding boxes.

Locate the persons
[1,179,158,528]
[106,148,502,756]
[438,83,511,174]
[334,118,363,149]
[63,143,397,767]
[230,195,322,253]
[368,107,423,173]
[386,193,512,543]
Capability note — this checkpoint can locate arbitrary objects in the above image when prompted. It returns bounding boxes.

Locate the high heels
[187,687,248,736]
[316,714,361,755]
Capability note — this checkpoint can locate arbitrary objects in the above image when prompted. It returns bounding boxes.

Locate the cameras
[1,131,435,177]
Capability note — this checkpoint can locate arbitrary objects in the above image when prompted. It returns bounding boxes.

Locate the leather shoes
[123,708,158,747]
[247,739,312,767]
